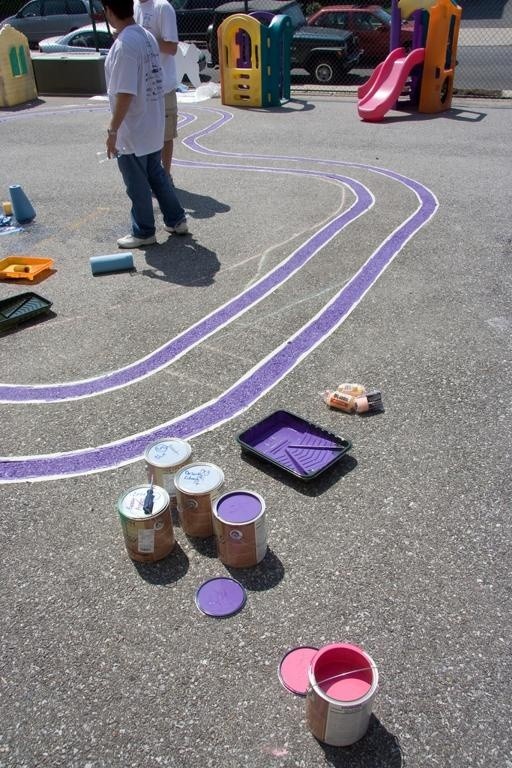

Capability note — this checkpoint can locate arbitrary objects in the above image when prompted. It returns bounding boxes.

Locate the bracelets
[106,128,120,135]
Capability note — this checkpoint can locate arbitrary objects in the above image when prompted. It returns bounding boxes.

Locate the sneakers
[117,234,156,249]
[164,223,188,234]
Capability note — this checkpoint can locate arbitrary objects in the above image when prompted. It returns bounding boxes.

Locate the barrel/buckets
[215,490,268,568]
[146,436,193,508]
[118,483,175,563]
[174,463,227,540]
[305,642,380,748]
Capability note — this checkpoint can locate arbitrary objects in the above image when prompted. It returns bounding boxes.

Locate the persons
[101,2,191,249]
[129,1,183,185]
